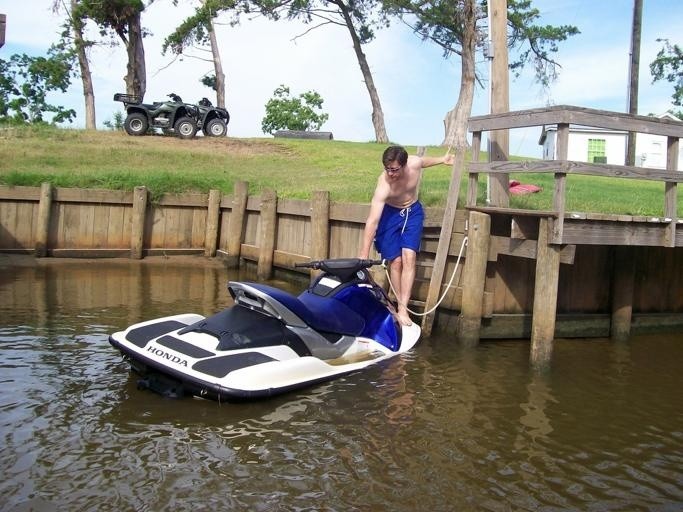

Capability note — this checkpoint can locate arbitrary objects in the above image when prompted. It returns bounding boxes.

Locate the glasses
[384,166,402,171]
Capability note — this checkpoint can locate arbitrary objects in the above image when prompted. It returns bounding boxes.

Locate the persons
[357,144,456,327]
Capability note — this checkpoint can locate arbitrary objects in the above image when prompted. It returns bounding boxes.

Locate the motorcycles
[153,97,232,139]
[104,258,420,404]
[110,89,202,140]
